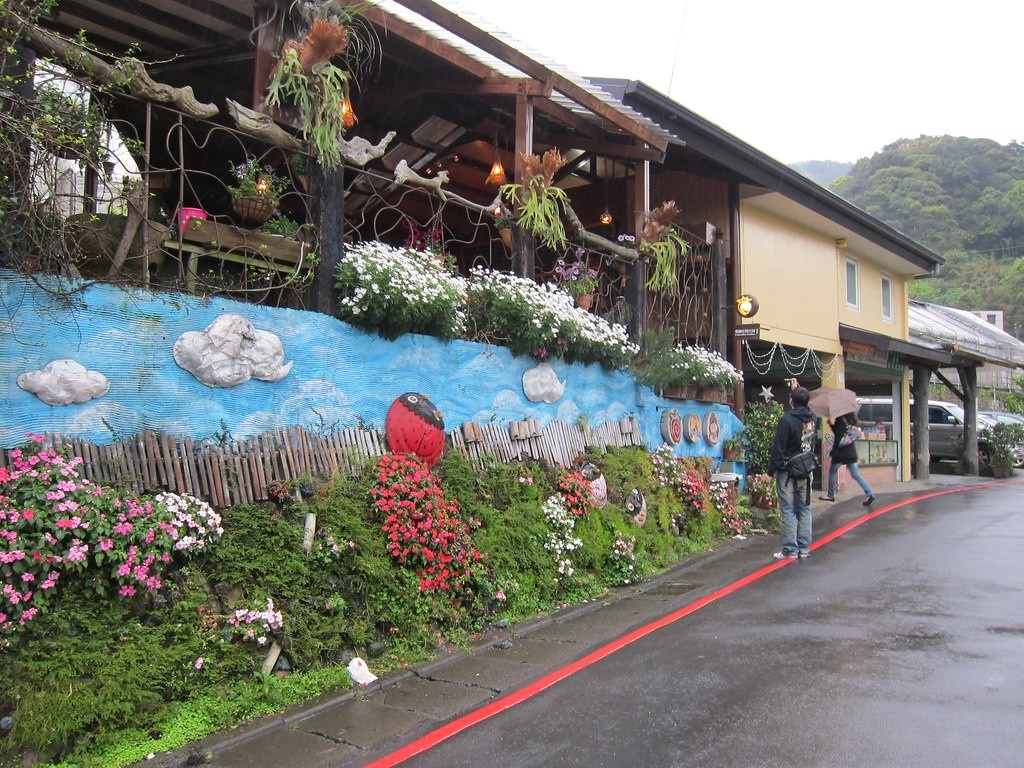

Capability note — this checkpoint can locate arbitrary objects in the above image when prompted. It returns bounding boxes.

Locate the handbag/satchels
[824,416,862,447]
[784,449,820,480]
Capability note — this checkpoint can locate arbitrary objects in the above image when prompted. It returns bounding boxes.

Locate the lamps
[600,160,614,223]
[486,131,507,187]
[342,81,359,128]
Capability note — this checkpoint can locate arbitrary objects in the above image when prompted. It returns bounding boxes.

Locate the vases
[696,388,728,403]
[755,494,778,509]
[664,384,697,400]
[576,291,593,313]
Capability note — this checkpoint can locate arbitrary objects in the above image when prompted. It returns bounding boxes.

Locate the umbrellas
[808,387,856,424]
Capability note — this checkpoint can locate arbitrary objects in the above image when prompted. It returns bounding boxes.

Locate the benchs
[160,214,311,298]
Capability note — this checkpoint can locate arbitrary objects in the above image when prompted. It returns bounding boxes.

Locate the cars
[978,411,1024,467]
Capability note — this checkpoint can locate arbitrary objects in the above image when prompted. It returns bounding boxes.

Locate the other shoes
[863,495,875,505]
[819,496,834,501]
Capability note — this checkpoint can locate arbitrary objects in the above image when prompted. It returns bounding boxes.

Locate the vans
[856,398,1003,467]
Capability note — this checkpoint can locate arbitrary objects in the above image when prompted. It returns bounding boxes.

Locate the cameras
[784,379,792,386]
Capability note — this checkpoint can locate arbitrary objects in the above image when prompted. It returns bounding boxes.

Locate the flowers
[555,246,598,293]
[746,473,774,509]
[227,153,294,208]
[339,238,469,343]
[232,196,275,224]
[649,343,748,390]
[470,265,643,376]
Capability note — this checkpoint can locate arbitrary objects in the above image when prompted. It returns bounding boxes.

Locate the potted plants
[638,199,691,291]
[266,17,353,171]
[722,438,738,461]
[102,122,116,178]
[66,178,170,284]
[500,147,577,254]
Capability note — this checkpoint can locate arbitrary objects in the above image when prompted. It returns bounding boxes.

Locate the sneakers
[773,550,808,559]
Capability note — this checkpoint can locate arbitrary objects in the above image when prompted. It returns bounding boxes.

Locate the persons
[819,413,876,505]
[761,378,817,559]
